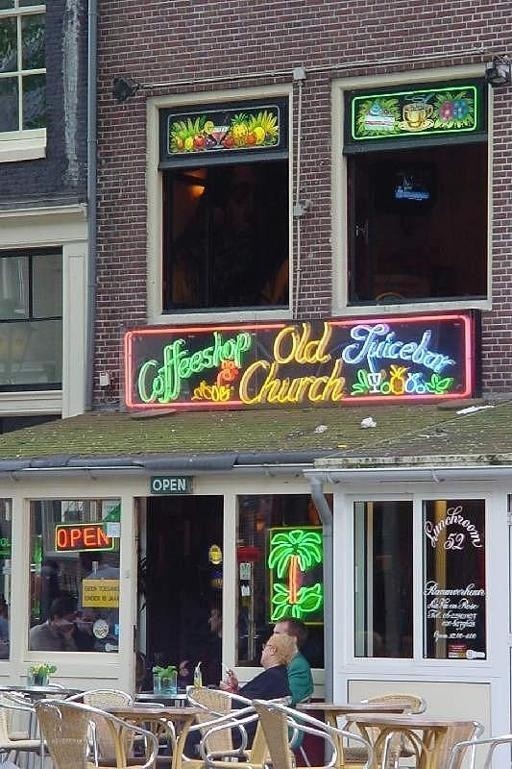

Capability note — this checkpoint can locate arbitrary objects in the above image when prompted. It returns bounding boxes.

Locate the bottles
[194,667,202,688]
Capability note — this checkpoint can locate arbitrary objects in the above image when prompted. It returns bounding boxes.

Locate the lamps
[483,54,509,87]
[111,74,141,104]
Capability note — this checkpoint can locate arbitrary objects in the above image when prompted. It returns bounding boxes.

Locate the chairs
[0,687,512,769]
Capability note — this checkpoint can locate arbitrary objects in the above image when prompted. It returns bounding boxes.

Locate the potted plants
[152,663,178,694]
[26,664,56,686]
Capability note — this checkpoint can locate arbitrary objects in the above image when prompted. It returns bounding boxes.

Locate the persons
[165,633,300,760]
[29,595,80,653]
[76,550,121,652]
[273,617,316,750]
[207,609,222,640]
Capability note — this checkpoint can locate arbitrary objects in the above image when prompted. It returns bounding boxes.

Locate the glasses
[262,643,274,649]
